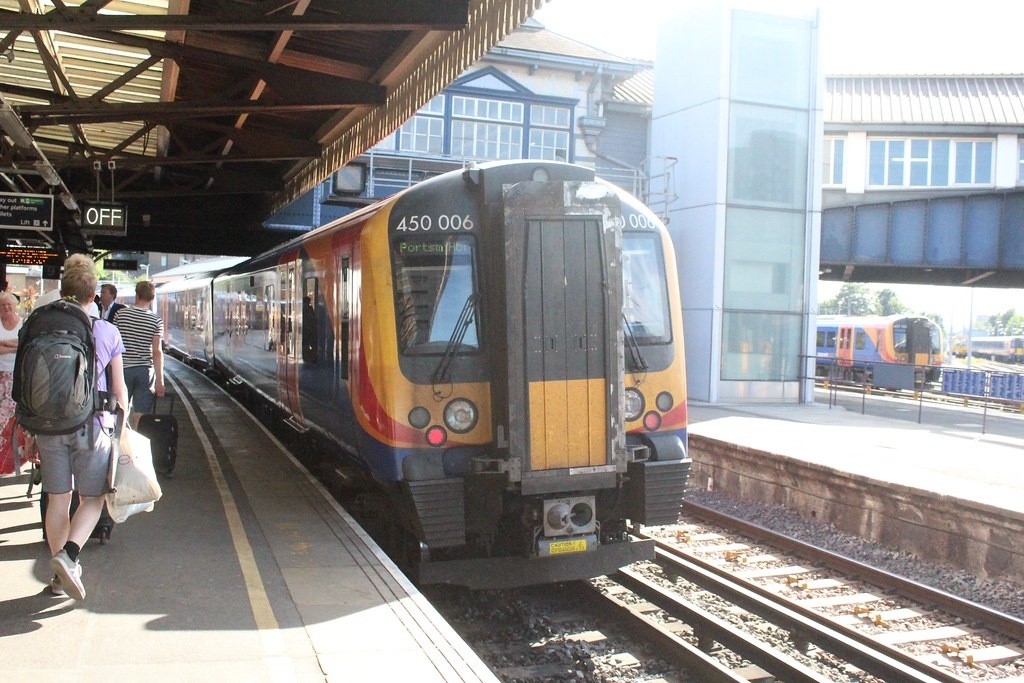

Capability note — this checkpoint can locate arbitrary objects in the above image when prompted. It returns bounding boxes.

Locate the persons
[0,294,30,433]
[112,281,165,432]
[97,284,127,324]
[34,253,100,318]
[37,269,129,600]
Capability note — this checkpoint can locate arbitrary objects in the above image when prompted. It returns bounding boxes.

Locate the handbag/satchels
[105,419,162,524]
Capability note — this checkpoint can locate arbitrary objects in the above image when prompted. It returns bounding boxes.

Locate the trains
[815,314,944,392]
[95,159,694,591]
[972,337,1024,363]
[949,333,972,360]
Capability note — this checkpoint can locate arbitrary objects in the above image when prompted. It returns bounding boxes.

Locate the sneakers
[49,550,87,600]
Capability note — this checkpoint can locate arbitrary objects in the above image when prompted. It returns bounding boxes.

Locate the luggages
[137,391,179,479]
[40,475,115,546]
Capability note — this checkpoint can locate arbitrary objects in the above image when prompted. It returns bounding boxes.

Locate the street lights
[140,264,149,280]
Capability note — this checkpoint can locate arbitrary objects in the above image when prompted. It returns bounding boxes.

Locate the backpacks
[12,296,109,498]
